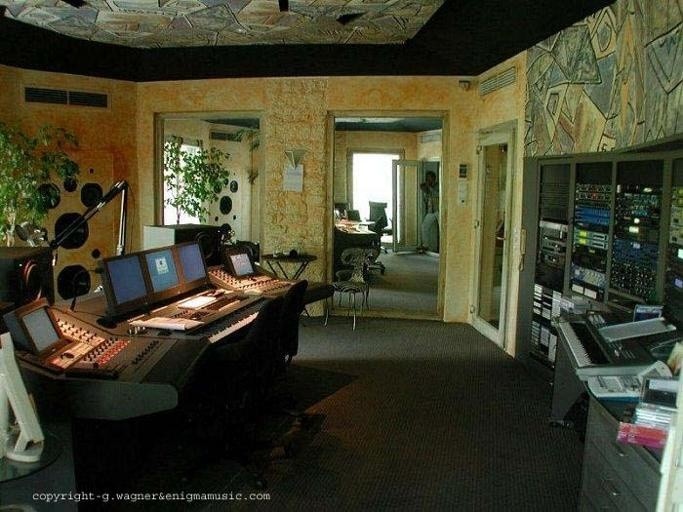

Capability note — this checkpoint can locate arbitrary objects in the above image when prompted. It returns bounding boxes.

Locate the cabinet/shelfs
[534,150,682,369]
[548,309,683,512]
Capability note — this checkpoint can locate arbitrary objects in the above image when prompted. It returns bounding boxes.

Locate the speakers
[1,246,56,305]
[143,224,220,264]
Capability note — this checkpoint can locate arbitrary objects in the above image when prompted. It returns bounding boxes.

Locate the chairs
[369,216,386,275]
[266,279,314,400]
[323,247,377,331]
[334,248,377,310]
[368,202,394,274]
[204,298,301,492]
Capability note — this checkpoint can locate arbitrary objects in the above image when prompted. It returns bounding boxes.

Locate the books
[616,402,677,450]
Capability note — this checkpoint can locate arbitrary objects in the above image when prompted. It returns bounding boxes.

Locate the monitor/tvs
[140,245,182,305]
[173,242,210,293]
[97,251,151,319]
[228,255,254,273]
[5,298,62,353]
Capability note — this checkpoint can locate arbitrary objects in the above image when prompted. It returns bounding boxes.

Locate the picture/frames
[0,330,62,462]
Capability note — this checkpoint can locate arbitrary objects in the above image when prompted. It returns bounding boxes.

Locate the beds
[259,250,318,327]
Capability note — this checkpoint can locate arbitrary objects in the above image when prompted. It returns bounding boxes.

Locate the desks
[0,258,308,512]
[333,217,376,276]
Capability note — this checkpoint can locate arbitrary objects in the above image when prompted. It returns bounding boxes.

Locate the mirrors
[151,110,262,269]
[323,108,447,322]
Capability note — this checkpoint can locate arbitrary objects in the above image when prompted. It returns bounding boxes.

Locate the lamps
[279,148,308,169]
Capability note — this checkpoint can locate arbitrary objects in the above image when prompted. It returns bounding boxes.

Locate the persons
[416,171,438,250]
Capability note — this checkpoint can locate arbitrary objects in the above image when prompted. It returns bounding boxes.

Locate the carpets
[82,361,358,512]
